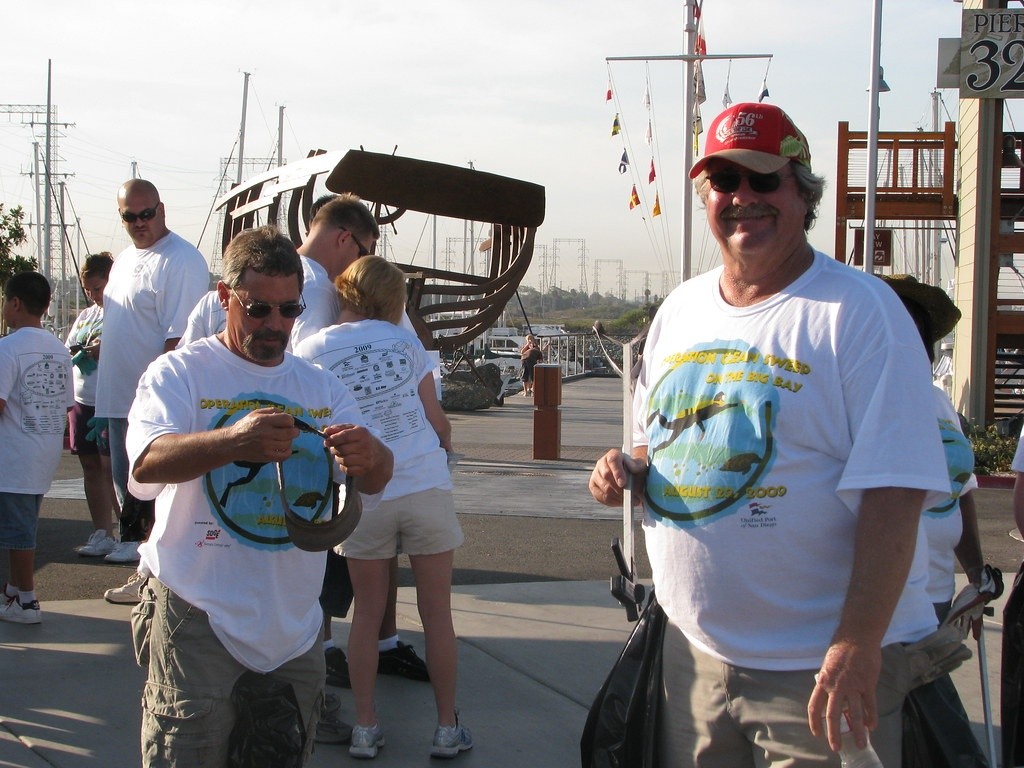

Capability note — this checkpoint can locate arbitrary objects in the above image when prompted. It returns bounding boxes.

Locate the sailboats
[407,161,582,397]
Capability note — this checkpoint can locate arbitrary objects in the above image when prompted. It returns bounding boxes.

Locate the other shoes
[523,389,535,397]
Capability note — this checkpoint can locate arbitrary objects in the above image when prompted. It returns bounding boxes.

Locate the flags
[692,6,706,157]
[758,77,770,103]
[721,82,733,109]
[604,79,641,211]
[639,82,661,218]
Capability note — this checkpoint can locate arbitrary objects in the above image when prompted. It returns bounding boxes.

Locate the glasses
[232,288,307,319]
[705,169,794,194]
[338,226,370,257]
[120,202,159,223]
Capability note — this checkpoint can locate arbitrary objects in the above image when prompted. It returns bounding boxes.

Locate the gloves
[941,564,1004,641]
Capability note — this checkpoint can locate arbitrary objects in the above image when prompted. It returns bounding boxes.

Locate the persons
[589,102,952,768]
[1010,424,1024,540]
[64,178,474,761]
[871,273,1005,768]
[126,225,395,768]
[520,334,543,397]
[1,271,75,625]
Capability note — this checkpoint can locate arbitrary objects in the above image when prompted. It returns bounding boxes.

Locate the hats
[276,417,363,552]
[873,273,962,343]
[688,102,813,179]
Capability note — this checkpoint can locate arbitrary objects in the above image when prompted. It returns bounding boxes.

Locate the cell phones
[70,345,83,352]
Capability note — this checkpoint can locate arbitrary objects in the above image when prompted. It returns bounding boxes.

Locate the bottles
[815,673,883,768]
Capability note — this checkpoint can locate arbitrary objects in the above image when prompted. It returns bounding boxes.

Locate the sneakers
[377,641,431,682]
[315,694,353,744]
[349,720,386,758]
[0,592,41,624]
[430,712,474,757]
[77,529,119,556]
[104,571,147,603]
[325,647,352,689]
[104,542,142,562]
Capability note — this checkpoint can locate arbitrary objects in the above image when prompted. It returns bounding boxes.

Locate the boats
[931,342,954,397]
